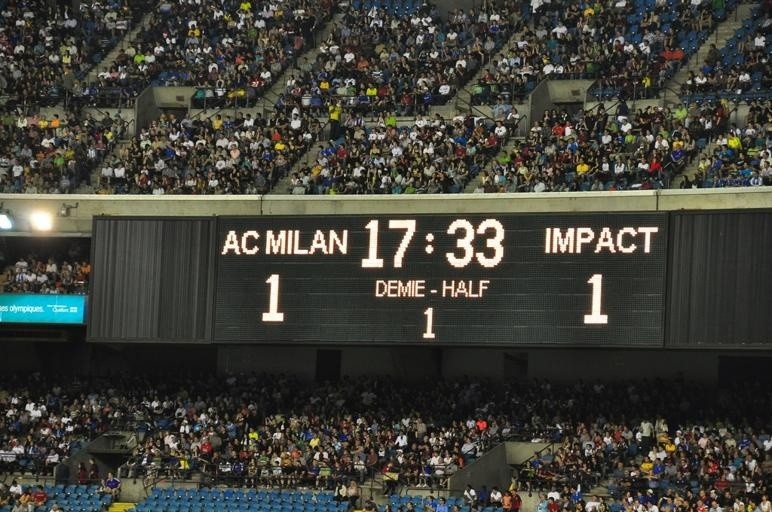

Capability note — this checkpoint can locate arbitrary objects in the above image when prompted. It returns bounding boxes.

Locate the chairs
[0,480,114,512]
[123,485,351,512]
[375,491,505,512]
[626,1,770,101]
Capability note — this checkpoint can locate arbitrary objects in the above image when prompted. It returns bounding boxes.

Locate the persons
[0,235,91,296]
